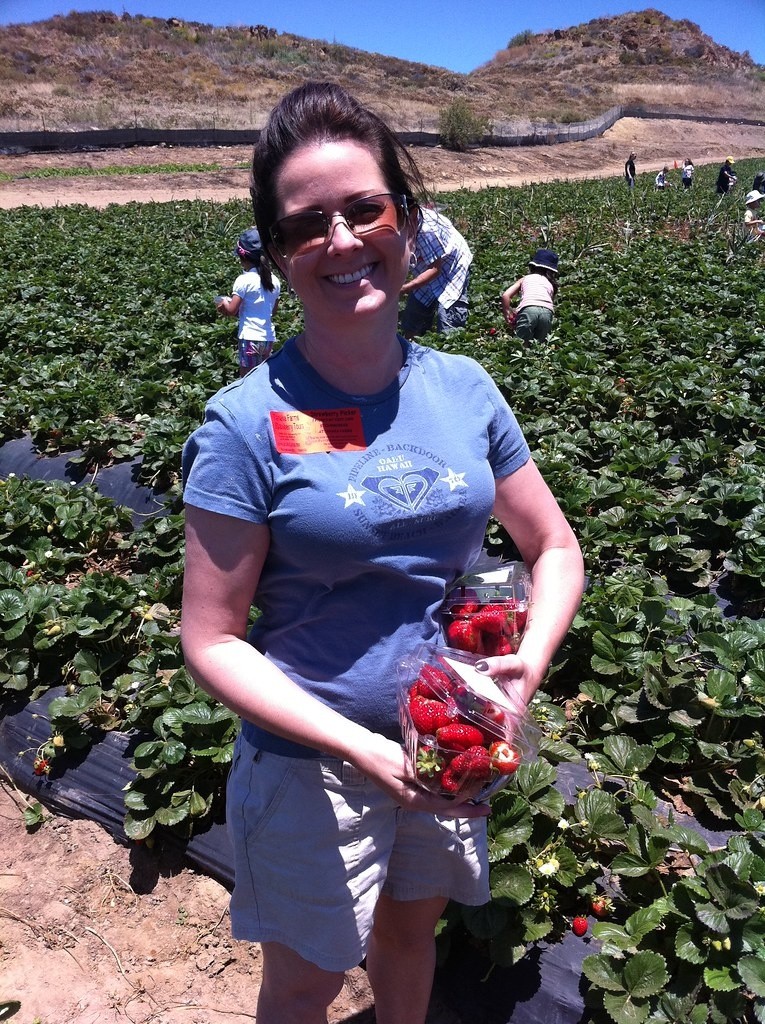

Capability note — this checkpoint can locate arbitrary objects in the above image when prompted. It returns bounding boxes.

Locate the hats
[725,155,734,163]
[527,249,561,274]
[664,165,669,170]
[744,190,765,203]
[236,228,263,257]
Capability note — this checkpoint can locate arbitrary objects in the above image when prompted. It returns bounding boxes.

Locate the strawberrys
[573,914,588,936]
[27,570,34,577]
[33,755,49,775]
[438,587,526,656]
[592,897,609,916]
[399,664,520,799]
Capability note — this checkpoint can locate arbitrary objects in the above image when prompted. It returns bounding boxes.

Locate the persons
[625,151,638,195]
[180,84,584,1024]
[502,251,559,339]
[217,229,280,378]
[401,206,471,339]
[656,167,669,191]
[682,158,695,189]
[753,173,765,195]
[716,156,736,193]
[743,191,765,238]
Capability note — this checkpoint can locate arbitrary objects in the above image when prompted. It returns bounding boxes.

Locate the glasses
[268,188,408,256]
